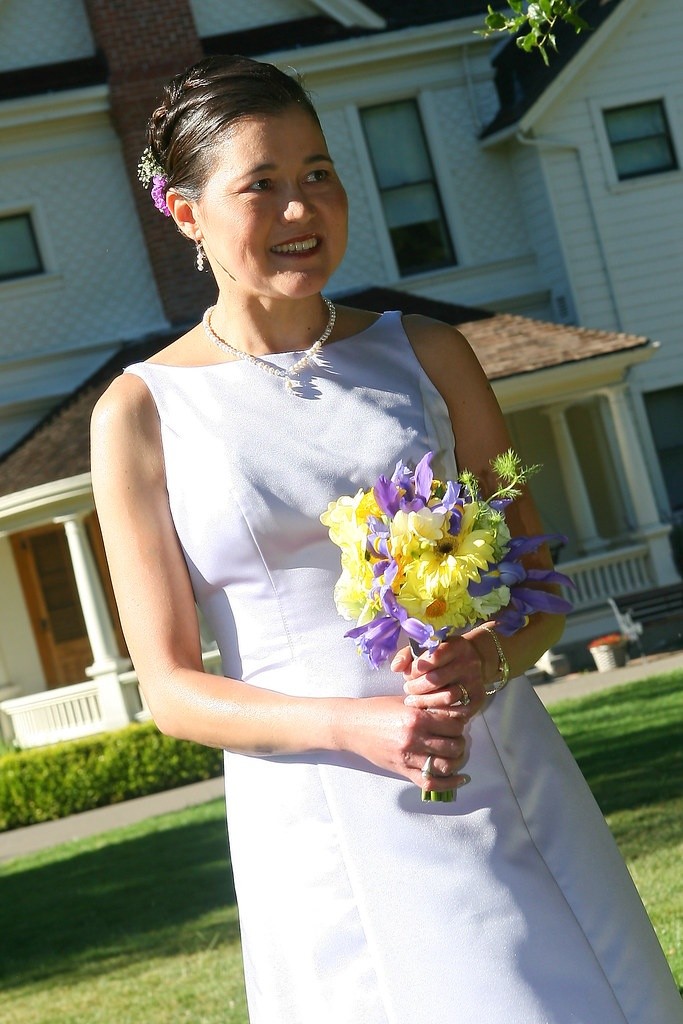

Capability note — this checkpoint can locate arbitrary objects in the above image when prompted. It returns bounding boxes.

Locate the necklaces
[201,294,339,397]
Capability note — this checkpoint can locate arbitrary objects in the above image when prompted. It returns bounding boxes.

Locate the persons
[90,52,681,1024]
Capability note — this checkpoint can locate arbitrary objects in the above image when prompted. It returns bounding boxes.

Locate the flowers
[318,448,577,803]
[586,633,627,648]
[136,146,170,218]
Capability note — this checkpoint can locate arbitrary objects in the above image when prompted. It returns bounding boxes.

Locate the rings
[420,754,434,781]
[458,682,471,706]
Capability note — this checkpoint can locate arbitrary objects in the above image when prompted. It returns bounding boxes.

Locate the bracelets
[469,625,511,696]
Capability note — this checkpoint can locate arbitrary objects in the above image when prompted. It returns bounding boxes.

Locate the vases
[589,643,625,672]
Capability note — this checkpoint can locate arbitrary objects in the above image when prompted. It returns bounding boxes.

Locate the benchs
[609,582,683,659]
